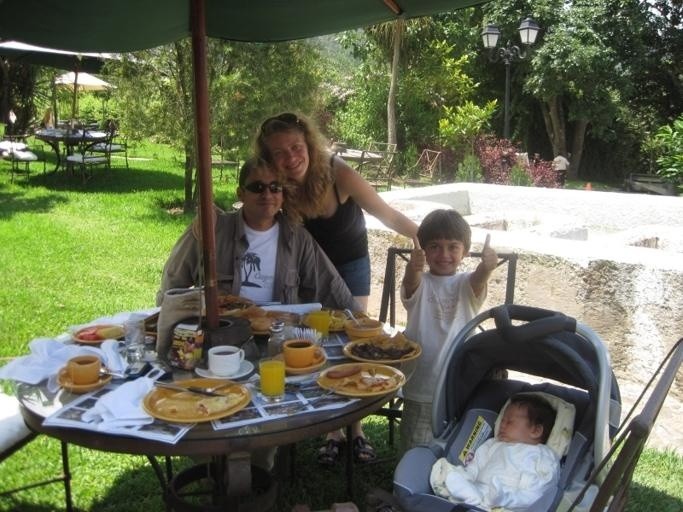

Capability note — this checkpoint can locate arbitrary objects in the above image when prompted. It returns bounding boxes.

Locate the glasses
[261,114,297,132]
[243,180,283,194]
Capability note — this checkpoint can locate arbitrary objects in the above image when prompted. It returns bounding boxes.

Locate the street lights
[480,20,543,138]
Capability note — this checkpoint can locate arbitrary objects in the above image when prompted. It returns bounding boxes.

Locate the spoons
[188,378,254,394]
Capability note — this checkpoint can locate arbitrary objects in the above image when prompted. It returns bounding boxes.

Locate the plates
[217,295,256,317]
[194,359,255,379]
[142,378,251,423]
[56,372,111,393]
[301,309,368,332]
[246,311,300,335]
[273,353,327,375]
[316,363,407,398]
[341,336,422,363]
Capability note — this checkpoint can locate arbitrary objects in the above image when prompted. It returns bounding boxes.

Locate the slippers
[316,435,346,467]
[347,437,376,463]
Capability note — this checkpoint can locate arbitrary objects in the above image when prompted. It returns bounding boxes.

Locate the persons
[399,208,499,470]
[251,112,428,466]
[430,393,561,508]
[152,153,368,474]
[499,146,572,189]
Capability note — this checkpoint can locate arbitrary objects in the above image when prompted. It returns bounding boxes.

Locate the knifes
[152,381,226,397]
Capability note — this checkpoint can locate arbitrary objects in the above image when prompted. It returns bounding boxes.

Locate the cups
[258,357,286,399]
[126,346,141,370]
[59,355,101,385]
[207,346,245,377]
[282,339,323,368]
[307,312,329,341]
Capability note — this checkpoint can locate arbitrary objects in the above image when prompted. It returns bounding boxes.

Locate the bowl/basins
[344,318,382,341]
[72,325,125,344]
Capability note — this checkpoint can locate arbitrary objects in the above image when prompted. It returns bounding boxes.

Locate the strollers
[393,304,681,507]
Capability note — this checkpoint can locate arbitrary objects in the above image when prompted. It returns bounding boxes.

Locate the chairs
[367,142,397,177]
[0,388,70,512]
[404,149,441,188]
[358,150,398,191]
[0,119,129,185]
[211,134,239,181]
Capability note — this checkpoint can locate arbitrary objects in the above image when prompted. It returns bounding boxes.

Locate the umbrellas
[2,0,496,328]
[0,39,139,127]
[48,71,120,96]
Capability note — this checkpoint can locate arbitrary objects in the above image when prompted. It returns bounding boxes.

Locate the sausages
[326,364,362,379]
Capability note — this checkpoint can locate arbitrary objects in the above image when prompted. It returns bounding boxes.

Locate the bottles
[266,321,285,359]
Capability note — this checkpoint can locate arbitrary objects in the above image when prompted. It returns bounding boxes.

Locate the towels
[0,338,91,385]
[261,303,322,314]
[95,377,155,427]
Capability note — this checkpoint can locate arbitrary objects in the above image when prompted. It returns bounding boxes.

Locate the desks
[333,149,383,165]
